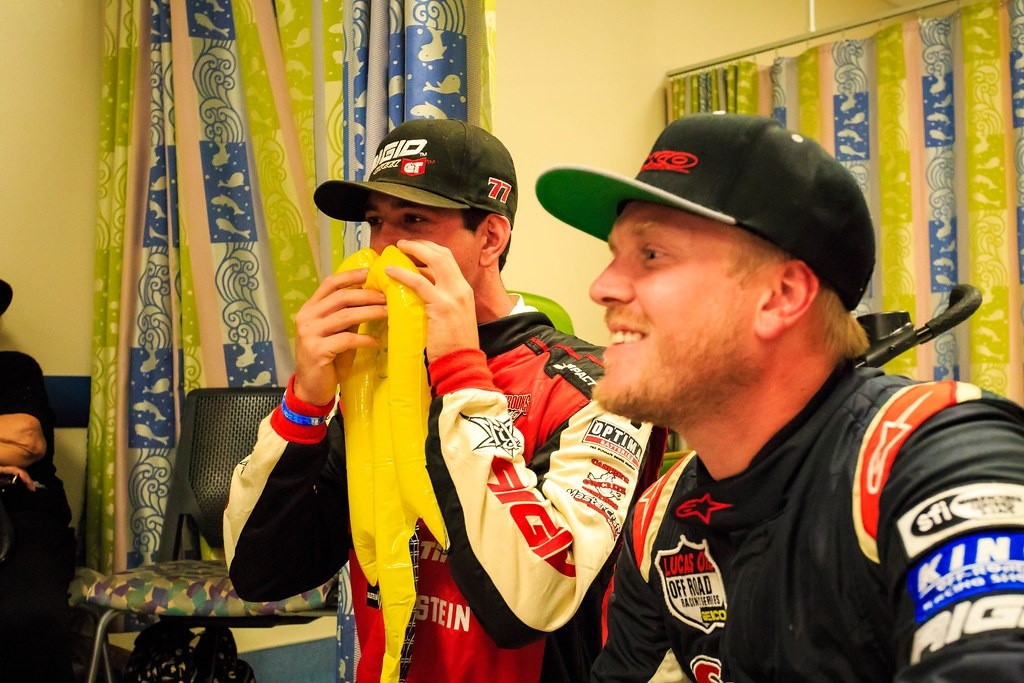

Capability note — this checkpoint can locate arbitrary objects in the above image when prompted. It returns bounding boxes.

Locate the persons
[224,122,670,683]
[592,113,1024,683]
[0,280,81,683]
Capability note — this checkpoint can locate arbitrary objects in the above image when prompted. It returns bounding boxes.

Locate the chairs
[84,390,338,683]
[43,375,115,683]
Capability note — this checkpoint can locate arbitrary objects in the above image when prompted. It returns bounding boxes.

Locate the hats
[533,112,878,312]
[312,119,519,222]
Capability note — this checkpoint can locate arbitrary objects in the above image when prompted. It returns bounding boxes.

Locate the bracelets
[278,387,331,426]
